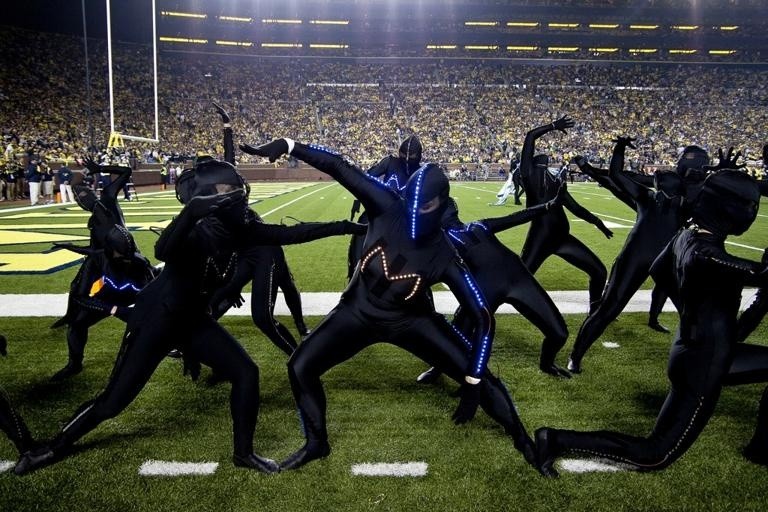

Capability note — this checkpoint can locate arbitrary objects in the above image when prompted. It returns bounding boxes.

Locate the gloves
[201,187,243,212]
[237,138,288,164]
[554,115,576,135]
[597,215,612,241]
[350,201,362,221]
[612,134,637,149]
[80,156,99,174]
[703,146,746,171]
[41,242,71,255]
[216,279,244,309]
[569,157,588,170]
[453,377,480,427]
[549,181,568,207]
[211,101,231,124]
[183,356,201,381]
[346,221,369,235]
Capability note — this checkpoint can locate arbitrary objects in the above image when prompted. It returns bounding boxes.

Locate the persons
[2,0,767,207]
[1,103,767,480]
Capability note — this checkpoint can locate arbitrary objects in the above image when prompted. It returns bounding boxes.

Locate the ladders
[94,131,139,202]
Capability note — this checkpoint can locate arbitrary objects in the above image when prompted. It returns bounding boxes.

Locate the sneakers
[540,354,581,377]
[418,368,441,383]
[282,443,331,471]
[526,427,560,480]
[50,363,83,381]
[233,452,278,474]
[13,439,65,476]
[52,316,70,330]
[651,319,671,334]
[168,348,183,358]
